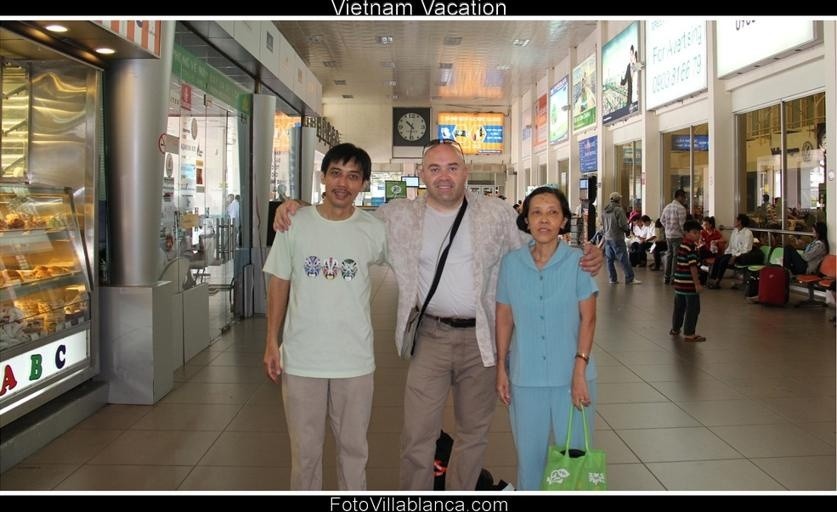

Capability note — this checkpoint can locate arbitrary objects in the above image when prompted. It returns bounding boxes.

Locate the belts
[415,305,476,328]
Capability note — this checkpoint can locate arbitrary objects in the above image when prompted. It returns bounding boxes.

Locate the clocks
[390,103,433,148]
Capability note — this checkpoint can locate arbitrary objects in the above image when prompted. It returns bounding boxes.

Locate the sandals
[669,329,681,335]
[684,334,706,343]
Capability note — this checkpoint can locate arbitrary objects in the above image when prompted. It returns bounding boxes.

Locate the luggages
[758,262,790,308]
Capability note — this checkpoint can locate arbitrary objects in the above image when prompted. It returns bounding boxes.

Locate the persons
[618,45,635,106]
[631,51,638,103]
[271,139,602,490]
[512,204,520,213]
[228,195,239,249]
[828,279,835,328]
[259,142,391,492]
[517,200,522,207]
[497,194,507,201]
[584,189,831,304]
[225,193,233,206]
[493,187,600,493]
[667,220,706,342]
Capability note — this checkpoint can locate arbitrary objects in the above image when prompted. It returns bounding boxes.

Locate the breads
[13,284,85,328]
[0,212,64,229]
[0,263,70,284]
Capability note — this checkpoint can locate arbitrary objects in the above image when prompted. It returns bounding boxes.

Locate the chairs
[183,233,219,297]
[648,232,837,328]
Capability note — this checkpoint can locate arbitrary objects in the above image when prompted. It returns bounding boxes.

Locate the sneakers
[609,281,620,284]
[664,277,673,285]
[625,278,643,285]
[631,262,660,271]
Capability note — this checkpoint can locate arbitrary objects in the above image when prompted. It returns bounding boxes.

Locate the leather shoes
[706,281,721,289]
[746,296,756,304]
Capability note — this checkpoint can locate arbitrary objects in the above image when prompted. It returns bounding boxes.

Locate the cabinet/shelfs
[0,180,102,430]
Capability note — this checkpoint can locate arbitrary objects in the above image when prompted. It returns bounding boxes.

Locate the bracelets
[574,350,590,364]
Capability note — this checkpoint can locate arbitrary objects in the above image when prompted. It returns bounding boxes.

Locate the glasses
[419,138,467,166]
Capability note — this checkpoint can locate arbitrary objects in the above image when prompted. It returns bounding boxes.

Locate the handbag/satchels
[538,399,608,490]
[400,305,419,361]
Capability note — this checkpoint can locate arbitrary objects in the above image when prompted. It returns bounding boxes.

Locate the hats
[627,210,640,221]
[609,191,623,202]
